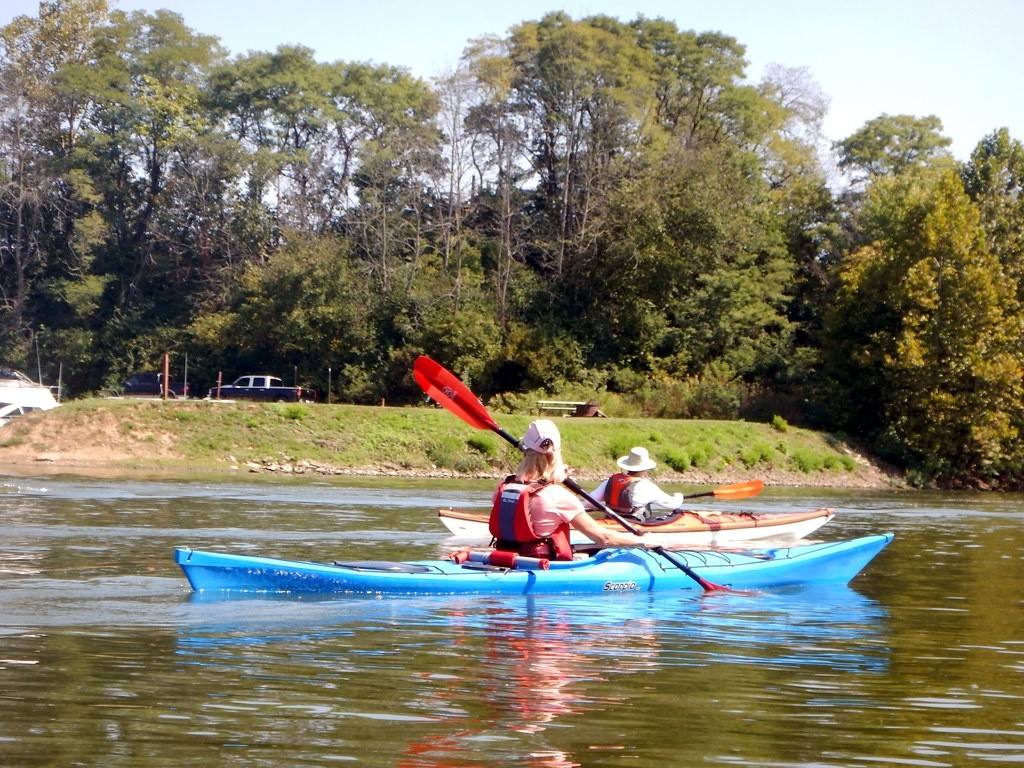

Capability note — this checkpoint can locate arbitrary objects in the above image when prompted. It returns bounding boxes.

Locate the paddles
[584,479,764,513]
[413,355,754,594]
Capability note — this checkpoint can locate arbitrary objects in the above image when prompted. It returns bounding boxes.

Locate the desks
[525,400,586,417]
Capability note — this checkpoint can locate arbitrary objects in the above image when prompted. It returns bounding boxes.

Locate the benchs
[526,407,576,411]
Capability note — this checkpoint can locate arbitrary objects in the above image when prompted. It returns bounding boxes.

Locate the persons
[584,447,683,522]
[488,419,662,560]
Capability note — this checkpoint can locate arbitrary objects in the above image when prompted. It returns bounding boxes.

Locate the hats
[616,447,657,472]
[522,418,561,454]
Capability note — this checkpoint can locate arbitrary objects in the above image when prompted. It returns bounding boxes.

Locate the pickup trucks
[209,374,318,406]
[121,371,191,395]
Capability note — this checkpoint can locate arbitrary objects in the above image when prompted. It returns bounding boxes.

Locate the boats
[170,530,897,604]
[438,504,834,545]
